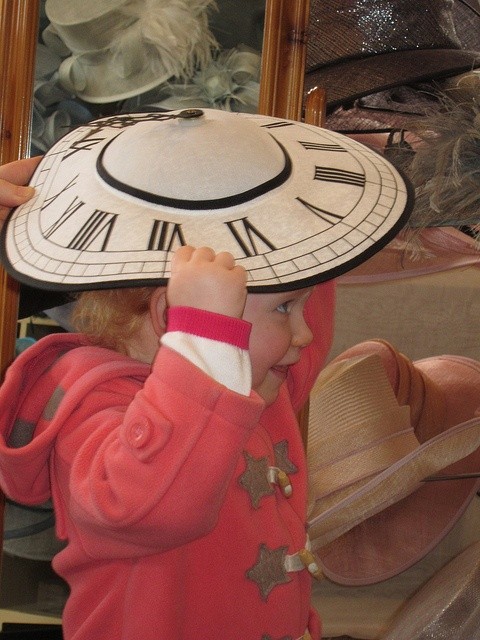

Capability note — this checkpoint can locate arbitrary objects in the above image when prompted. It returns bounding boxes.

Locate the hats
[313,340,480,588]
[2,105,413,292]
[321,126,478,286]
[306,356,480,549]
[305,0,478,108]
[42,1,208,104]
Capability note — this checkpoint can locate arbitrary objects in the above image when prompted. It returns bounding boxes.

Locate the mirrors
[1,1,326,405]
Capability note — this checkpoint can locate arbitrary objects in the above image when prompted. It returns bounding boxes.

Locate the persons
[0,154,49,223]
[0,108,414,640]
